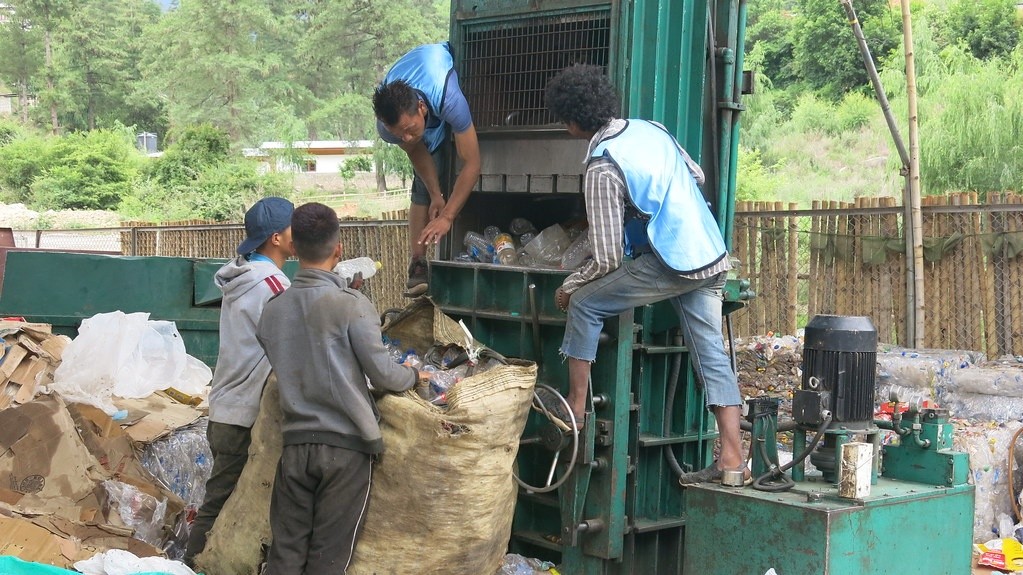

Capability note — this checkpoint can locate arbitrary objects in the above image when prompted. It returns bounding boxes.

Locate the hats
[236,196,294,254]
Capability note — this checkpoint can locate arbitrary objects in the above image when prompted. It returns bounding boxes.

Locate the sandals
[679,459,753,486]
[532,387,585,435]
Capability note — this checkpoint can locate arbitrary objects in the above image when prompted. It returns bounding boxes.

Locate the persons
[543,65,754,487]
[255,202,432,575]
[372,42,481,295]
[183,196,364,571]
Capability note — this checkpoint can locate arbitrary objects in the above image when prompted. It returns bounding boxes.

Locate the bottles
[334,257,381,283]
[715,332,1023,548]
[456,218,592,271]
[140,417,215,564]
[381,334,503,405]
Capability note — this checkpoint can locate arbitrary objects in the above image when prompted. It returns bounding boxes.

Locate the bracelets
[559,285,567,313]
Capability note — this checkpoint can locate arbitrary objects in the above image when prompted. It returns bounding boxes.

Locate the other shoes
[406,260,428,297]
